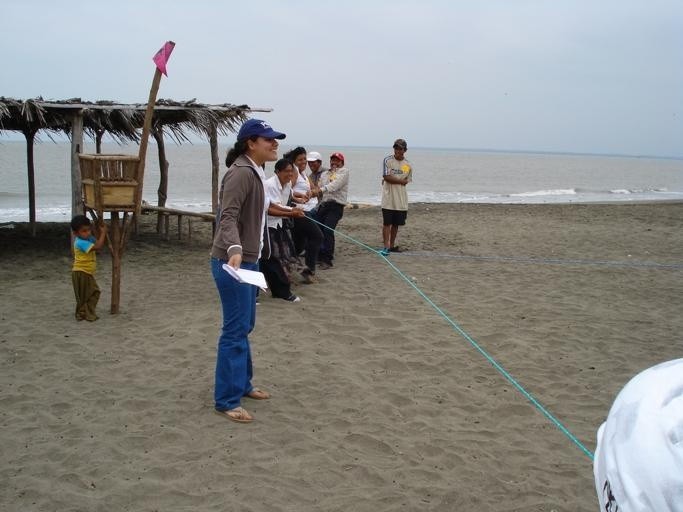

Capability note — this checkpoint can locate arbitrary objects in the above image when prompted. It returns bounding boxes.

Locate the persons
[255,146,350,305]
[70,215,110,322]
[380,138,412,256]
[210,119,287,424]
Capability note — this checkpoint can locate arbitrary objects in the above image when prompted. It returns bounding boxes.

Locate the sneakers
[380,246,399,256]
[301,262,333,283]
[284,293,301,303]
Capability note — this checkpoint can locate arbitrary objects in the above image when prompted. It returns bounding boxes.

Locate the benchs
[141,202,216,249]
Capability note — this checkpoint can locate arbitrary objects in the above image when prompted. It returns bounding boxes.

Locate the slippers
[248,387,271,399]
[212,406,252,423]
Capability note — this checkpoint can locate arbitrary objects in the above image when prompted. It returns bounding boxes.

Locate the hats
[392,138,407,149]
[330,152,343,161]
[237,118,286,140]
[306,151,322,161]
